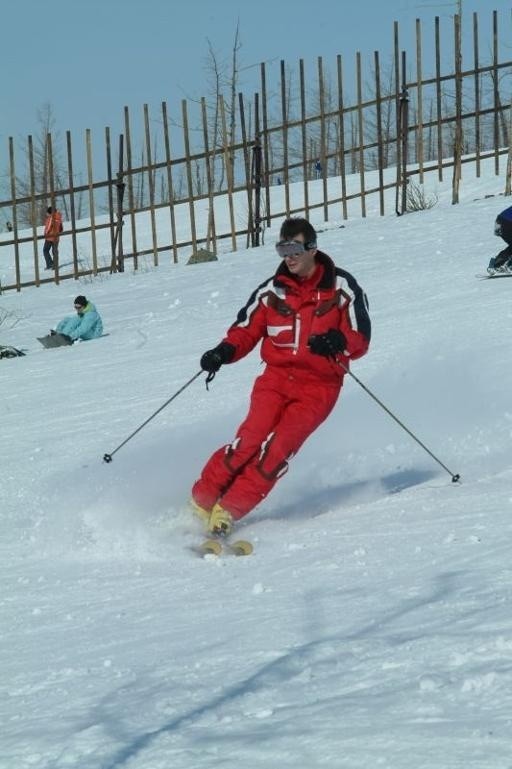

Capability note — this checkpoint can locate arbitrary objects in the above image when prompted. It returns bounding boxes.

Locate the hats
[74,295,86,306]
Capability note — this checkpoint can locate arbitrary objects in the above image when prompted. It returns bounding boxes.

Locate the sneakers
[189,499,211,521]
[206,503,234,538]
[487,266,507,274]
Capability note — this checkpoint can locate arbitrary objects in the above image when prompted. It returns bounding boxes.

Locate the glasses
[276,240,316,257]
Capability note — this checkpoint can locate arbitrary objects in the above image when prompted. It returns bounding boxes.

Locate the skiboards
[476,275,511,280]
[183,542,253,559]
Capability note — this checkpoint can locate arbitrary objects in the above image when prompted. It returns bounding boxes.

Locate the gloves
[200,342,237,390]
[307,328,347,357]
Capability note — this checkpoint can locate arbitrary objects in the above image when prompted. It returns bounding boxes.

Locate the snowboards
[37,334,72,348]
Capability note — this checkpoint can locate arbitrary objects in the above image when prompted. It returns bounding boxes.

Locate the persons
[188,216,374,539]
[50,293,103,343]
[42,206,64,270]
[486,205,511,274]
